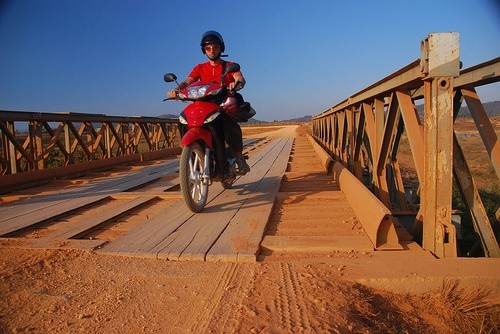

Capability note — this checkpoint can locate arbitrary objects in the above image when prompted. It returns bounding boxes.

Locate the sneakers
[236,157,250,173]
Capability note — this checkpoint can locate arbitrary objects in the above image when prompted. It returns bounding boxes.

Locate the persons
[166,30,252,174]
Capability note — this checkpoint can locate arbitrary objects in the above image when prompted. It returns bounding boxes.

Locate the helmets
[200,31,225,55]
[220,93,256,122]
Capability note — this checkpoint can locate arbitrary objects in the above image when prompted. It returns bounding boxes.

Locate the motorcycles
[163,62,250,213]
